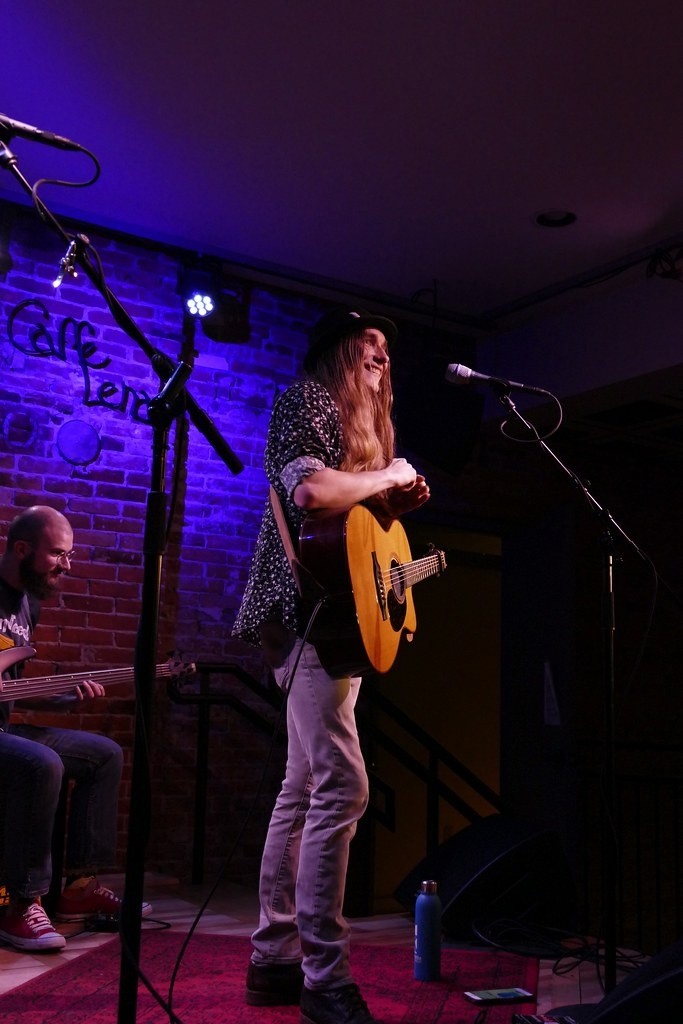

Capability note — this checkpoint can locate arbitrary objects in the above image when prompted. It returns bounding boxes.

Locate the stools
[0,743,76,920]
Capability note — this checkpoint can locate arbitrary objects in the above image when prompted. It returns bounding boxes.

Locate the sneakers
[300,980,381,1023]
[246,958,309,1007]
[0,897,66,949]
[56,880,153,921]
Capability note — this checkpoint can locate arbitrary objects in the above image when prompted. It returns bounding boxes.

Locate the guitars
[0,645,194,732]
[298,504,448,682]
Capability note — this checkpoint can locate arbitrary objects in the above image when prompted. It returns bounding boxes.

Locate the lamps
[184,281,217,319]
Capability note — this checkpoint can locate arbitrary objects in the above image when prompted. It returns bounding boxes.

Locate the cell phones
[461,986,535,1005]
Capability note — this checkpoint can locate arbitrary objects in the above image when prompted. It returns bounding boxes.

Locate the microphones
[445,363,551,399]
[0,113,81,152]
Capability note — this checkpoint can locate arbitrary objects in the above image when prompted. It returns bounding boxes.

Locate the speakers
[580,942,683,1024]
[393,815,578,954]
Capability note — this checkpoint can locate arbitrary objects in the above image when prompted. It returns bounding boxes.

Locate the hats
[301,306,398,375]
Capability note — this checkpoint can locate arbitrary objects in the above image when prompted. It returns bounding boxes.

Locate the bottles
[413,880,441,981]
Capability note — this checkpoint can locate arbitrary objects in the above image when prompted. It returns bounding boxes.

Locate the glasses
[30,542,75,564]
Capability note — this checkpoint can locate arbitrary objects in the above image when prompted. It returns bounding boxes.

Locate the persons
[231,309,431,1024]
[0,505,154,949]
